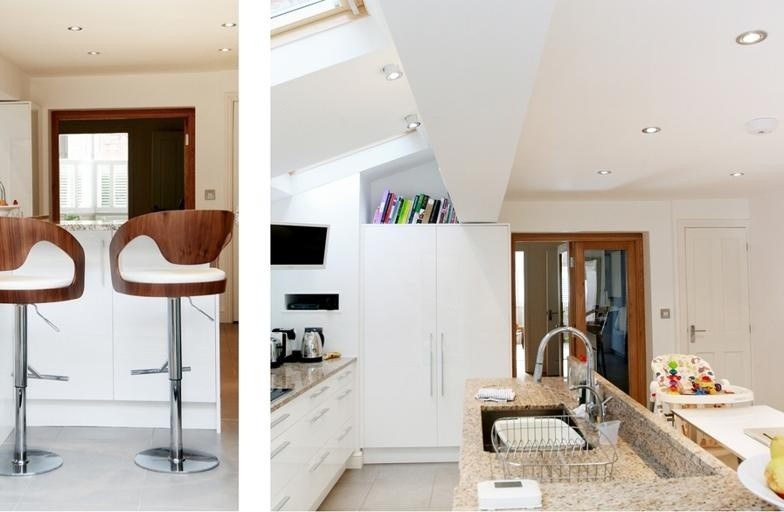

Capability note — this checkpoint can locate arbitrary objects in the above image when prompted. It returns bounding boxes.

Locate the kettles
[301,326,325,362]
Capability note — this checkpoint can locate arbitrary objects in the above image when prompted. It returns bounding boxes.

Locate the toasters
[269,337,283,369]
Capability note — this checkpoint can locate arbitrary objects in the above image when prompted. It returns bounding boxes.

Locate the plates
[734,451,784,511]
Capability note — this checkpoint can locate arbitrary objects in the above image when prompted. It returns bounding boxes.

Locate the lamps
[402,111,421,132]
[383,60,404,81]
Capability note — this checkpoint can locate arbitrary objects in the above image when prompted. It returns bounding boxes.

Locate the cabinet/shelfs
[0,101,40,214]
[331,366,360,485]
[272,397,314,511]
[307,383,340,508]
[356,223,510,465]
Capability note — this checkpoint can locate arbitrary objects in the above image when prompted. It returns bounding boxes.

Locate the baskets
[490,415,619,484]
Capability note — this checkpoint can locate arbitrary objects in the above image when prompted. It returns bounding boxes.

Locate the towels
[472,385,517,402]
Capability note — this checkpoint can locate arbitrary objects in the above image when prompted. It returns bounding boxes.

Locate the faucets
[570,380,603,424]
[534,326,599,426]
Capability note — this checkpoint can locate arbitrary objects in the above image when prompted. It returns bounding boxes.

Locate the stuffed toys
[665,357,735,395]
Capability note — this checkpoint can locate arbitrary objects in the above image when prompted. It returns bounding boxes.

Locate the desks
[657,382,754,406]
[670,404,784,462]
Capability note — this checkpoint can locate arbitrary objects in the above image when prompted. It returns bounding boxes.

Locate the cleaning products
[576,352,587,405]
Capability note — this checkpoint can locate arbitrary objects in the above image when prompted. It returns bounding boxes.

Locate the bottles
[0,180,6,206]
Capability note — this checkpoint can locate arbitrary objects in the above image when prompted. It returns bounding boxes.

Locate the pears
[763,434,784,499]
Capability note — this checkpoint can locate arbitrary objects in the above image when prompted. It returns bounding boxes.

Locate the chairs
[107,208,237,474]
[516,322,525,348]
[0,215,87,479]
[649,356,758,465]
[1,232,223,437]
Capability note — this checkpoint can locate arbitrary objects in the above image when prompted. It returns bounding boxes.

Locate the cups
[598,419,621,446]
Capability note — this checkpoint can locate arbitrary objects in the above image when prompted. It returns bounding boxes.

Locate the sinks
[481,402,594,452]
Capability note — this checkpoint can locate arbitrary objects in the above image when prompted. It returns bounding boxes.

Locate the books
[371,185,459,224]
